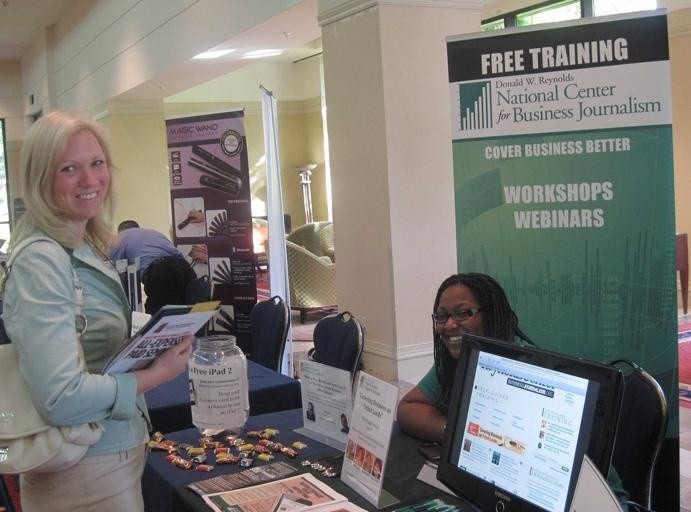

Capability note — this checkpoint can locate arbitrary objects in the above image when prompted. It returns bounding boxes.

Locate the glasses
[432,306,481,323]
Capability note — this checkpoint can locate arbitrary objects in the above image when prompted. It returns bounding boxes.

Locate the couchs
[320,223,339,255]
[286,237,337,324]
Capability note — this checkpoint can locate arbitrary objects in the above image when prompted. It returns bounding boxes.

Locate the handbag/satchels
[0,234,102,476]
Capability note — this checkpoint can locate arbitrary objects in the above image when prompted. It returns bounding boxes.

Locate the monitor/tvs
[436,333,626,512]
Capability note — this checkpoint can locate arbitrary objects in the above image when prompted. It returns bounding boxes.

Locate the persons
[106,219,198,317]
[2,111,196,512]
[396,274,541,443]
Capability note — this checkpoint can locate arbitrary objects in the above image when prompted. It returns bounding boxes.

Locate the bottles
[185,337,249,433]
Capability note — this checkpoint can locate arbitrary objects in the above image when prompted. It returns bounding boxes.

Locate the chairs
[607,358,669,509]
[244,295,291,374]
[308,311,365,392]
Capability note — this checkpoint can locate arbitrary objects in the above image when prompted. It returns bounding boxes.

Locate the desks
[143,357,301,410]
[147,410,477,510]
